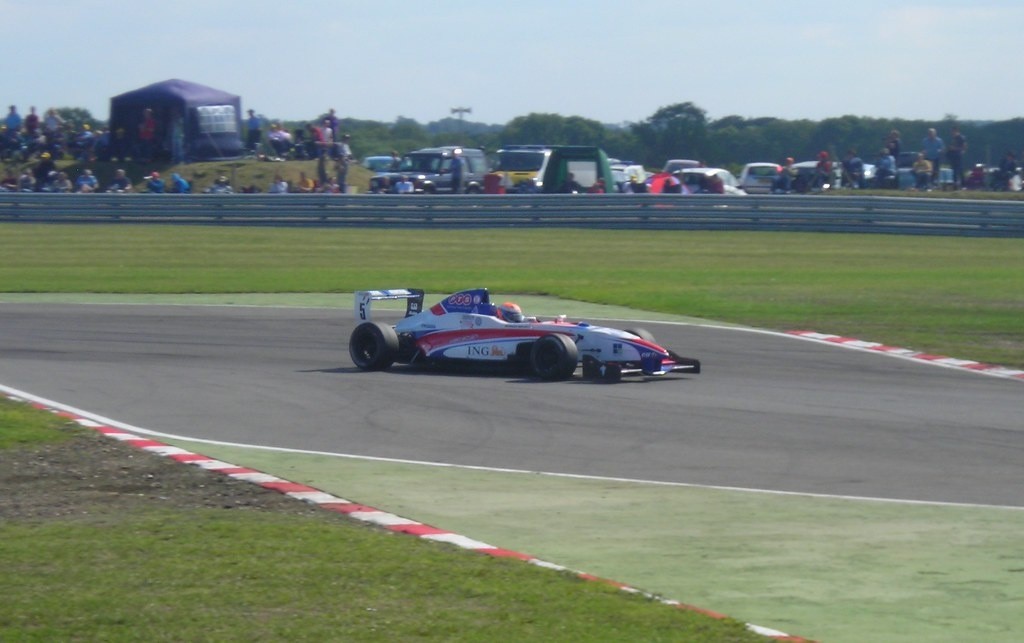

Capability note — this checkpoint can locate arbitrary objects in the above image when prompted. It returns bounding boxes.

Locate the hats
[151,171,159,176]
[817,151,828,158]
[220,175,226,182]
[786,157,794,163]
[248,109,254,113]
[83,123,90,130]
[40,152,51,158]
[270,124,277,129]
[631,174,637,181]
[776,166,783,172]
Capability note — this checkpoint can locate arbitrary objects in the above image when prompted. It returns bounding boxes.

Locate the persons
[510,171,606,193]
[0,106,358,194]
[497,302,542,324]
[624,174,725,194]
[367,148,464,194]
[776,127,1024,194]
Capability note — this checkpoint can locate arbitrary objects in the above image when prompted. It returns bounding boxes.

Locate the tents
[640,172,691,194]
[109,79,242,161]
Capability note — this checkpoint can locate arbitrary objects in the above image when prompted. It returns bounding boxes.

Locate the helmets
[497,302,523,323]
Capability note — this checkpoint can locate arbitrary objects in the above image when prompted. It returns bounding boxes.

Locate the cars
[367,136,956,195]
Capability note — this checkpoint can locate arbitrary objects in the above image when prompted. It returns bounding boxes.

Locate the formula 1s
[349,286,703,384]
